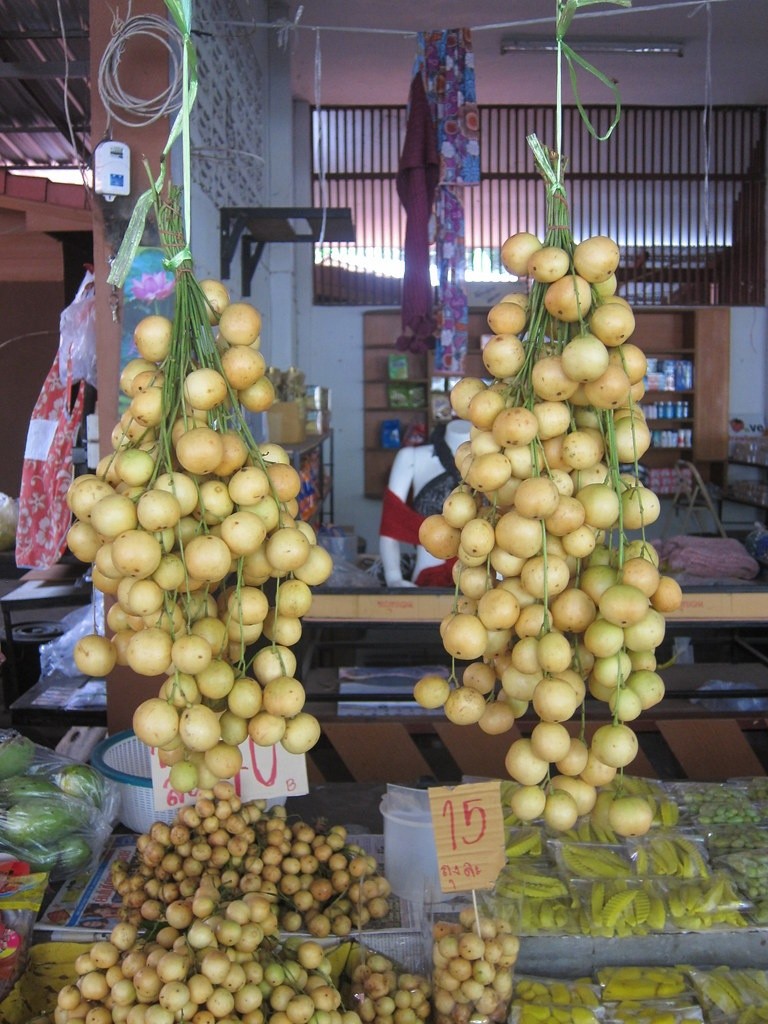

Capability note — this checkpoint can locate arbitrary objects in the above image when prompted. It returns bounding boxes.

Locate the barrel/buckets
[380,802,457,902]
[318,526,357,568]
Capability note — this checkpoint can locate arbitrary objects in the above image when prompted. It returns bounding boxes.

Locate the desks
[274,431,335,534]
[280,583,768,657]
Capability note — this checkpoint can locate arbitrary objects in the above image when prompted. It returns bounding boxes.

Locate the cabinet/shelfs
[362,303,730,506]
[0,191,191,736]
[714,458,768,539]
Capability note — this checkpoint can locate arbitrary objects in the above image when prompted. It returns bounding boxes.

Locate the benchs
[8,659,768,781]
